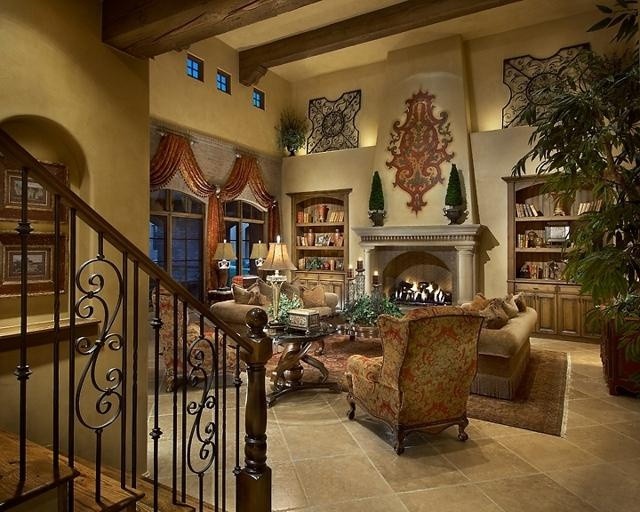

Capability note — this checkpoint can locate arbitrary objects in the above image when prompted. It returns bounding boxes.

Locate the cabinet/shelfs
[500,175,600,343]
[285,188,352,313]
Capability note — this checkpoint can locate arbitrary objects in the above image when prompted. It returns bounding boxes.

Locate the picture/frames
[1,156,75,298]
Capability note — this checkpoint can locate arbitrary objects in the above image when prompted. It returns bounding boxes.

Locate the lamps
[212,235,298,328]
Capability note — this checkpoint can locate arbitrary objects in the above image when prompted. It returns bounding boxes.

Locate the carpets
[266,335,570,438]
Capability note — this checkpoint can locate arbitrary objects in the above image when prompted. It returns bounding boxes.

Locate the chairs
[152,287,241,392]
[461,306,539,400]
[346,308,485,455]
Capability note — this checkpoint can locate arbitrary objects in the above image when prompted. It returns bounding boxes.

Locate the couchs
[210,293,340,334]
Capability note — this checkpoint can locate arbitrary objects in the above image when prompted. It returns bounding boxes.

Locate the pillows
[231,279,329,308]
[461,292,526,330]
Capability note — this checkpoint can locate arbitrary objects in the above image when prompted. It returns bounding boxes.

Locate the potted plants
[273,105,313,157]
[368,171,385,227]
[444,167,464,225]
[510,0,640,399]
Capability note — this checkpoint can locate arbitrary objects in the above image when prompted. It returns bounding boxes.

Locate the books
[577,198,604,215]
[517,233,529,249]
[515,203,539,218]
[529,261,560,280]
